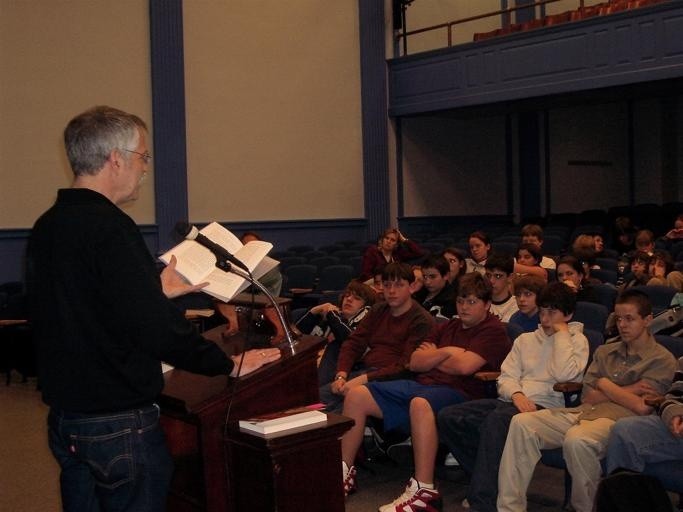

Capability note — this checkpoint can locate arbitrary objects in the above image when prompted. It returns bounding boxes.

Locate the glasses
[123,147,153,165]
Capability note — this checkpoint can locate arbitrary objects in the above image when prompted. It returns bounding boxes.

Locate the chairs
[472,0,670,43]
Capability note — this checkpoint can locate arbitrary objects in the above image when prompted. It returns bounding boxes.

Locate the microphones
[172,217,249,269]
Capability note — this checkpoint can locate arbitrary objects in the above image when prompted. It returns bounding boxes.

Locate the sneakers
[379,478,442,512]
[342,460,357,500]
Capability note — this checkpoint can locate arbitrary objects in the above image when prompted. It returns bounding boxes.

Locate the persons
[241,233,284,333]
[295,214,683,512]
[26,108,282,512]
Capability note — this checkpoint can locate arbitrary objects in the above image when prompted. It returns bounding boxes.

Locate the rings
[262,352,265,356]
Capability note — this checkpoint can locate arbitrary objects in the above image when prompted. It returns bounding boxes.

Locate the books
[157,221,281,304]
[238,402,328,434]
